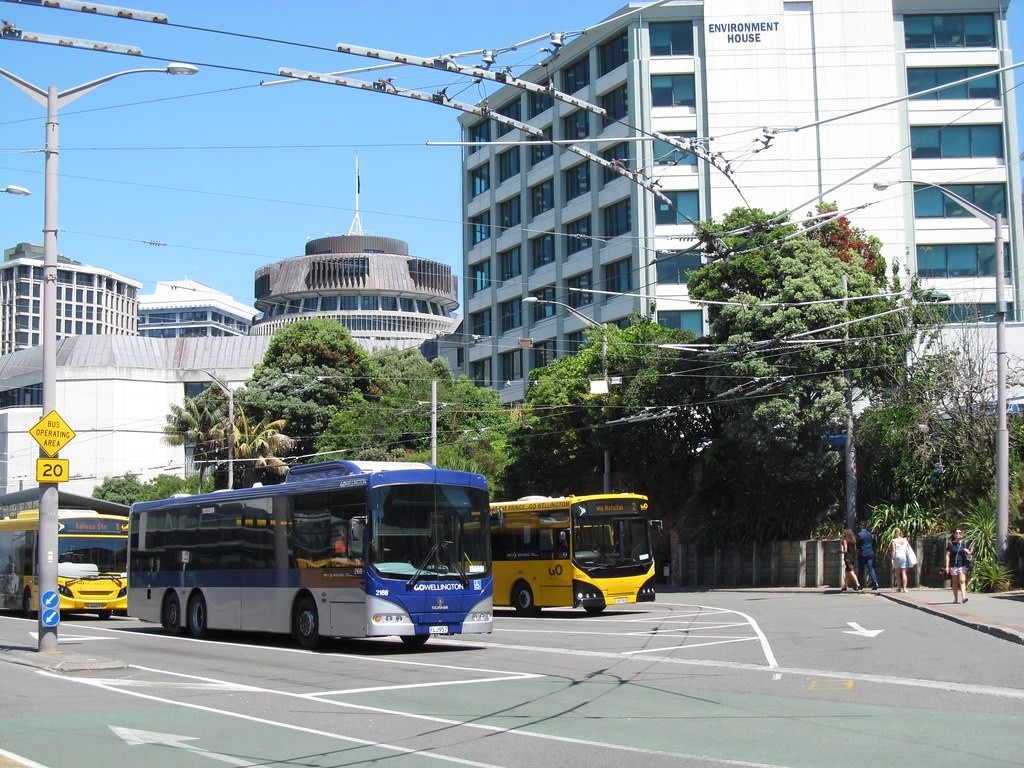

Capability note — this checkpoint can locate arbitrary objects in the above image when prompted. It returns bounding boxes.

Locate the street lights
[0,62,203,659]
[519,294,613,496]
[183,365,238,490]
[877,179,1013,567]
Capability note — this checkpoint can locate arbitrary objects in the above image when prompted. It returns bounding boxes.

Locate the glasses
[956,532,963,535]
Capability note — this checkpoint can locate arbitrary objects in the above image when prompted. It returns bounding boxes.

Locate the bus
[488,492,657,617]
[0,510,130,624]
[126,466,494,650]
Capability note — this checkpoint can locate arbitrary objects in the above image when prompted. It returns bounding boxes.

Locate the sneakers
[962,597,969,603]
[895,589,901,592]
[856,586,862,590]
[872,584,877,590]
[842,587,848,591]
[953,600,958,603]
[903,590,909,593]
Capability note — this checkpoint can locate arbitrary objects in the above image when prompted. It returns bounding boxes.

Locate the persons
[856,521,879,590]
[836,529,862,591]
[946,530,972,603]
[891,528,908,593]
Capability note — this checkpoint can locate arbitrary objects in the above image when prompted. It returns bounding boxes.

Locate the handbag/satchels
[942,568,951,580]
[964,540,974,569]
[907,542,918,568]
